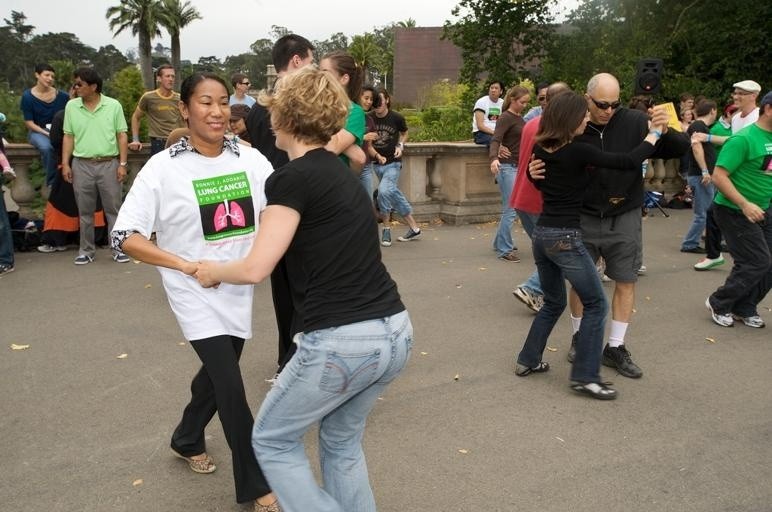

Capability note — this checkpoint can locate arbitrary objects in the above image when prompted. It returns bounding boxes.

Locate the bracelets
[133,136,139,139]
[120,162,129,167]
[650,131,661,137]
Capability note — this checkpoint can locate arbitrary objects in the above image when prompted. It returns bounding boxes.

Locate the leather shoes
[681,246,706,254]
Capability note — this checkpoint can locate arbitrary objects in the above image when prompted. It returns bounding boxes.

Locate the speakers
[635,59,663,94]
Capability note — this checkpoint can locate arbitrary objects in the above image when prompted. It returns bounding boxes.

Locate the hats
[732,80,762,93]
[230,104,250,121]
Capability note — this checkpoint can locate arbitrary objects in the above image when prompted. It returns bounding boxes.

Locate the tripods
[643,190,669,217]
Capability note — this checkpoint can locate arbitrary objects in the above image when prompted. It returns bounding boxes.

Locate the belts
[78,157,114,162]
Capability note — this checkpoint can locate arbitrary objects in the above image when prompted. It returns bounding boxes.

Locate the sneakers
[170,447,216,473]
[695,252,724,270]
[500,254,520,262]
[2,167,16,181]
[112,248,130,263]
[255,497,280,512]
[0,264,14,275]
[381,227,392,246]
[705,296,765,328]
[397,227,420,241]
[514,362,549,376]
[74,254,96,264]
[37,243,68,252]
[567,330,643,399]
[513,286,544,312]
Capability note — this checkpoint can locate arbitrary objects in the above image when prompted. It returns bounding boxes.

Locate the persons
[516,92,667,399]
[526,72,691,377]
[62,67,129,266]
[193,70,415,512]
[109,72,279,512]
[679,79,760,271]
[128,64,187,158]
[20,63,107,252]
[472,79,656,312]
[705,91,772,330]
[230,35,421,246]
[1,113,17,275]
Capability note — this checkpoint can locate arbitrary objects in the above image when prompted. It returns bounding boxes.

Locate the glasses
[591,98,621,109]
[240,83,249,85]
[539,97,545,101]
